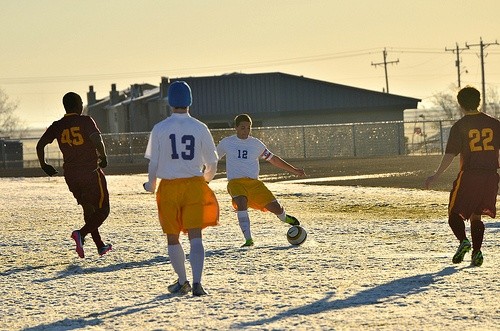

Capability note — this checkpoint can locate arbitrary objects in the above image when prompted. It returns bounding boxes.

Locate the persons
[35,92,113,258]
[142,82,219,294]
[217,113,307,247]
[426,87,500,268]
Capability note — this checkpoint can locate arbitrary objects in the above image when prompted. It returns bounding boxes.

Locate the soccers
[287,225,307,245]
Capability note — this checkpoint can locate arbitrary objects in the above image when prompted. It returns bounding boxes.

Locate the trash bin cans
[0,139,24,177]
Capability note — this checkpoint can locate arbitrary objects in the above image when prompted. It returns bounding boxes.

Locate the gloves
[40,163,59,177]
[97,156,108,169]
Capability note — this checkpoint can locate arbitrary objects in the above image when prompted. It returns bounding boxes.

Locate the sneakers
[452,238,472,264]
[167,278,191,294]
[240,238,255,248]
[70,229,86,258]
[97,244,112,257]
[277,213,301,226]
[470,247,484,267]
[191,282,207,297]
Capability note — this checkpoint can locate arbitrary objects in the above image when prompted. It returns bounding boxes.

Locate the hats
[167,81,193,108]
